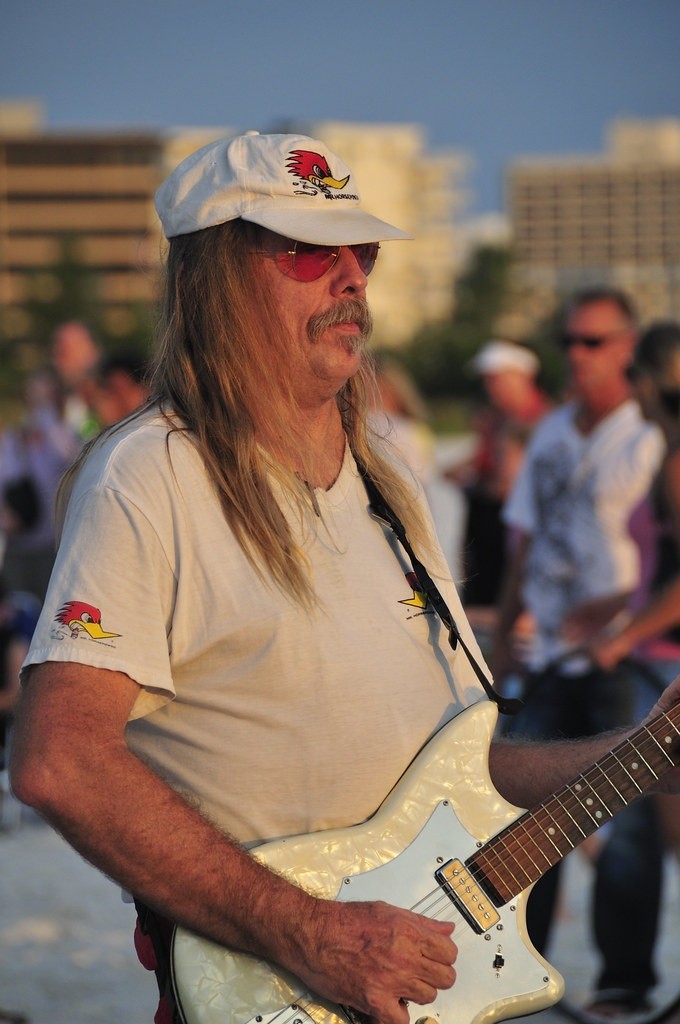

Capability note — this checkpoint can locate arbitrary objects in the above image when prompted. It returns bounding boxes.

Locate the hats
[465,339,542,378]
[153,129,415,246]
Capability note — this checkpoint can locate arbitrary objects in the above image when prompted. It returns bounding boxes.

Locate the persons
[366,355,437,485]
[558,323,680,1015]
[463,334,553,609]
[0,319,153,713]
[8,131,680,1024]
[499,283,667,957]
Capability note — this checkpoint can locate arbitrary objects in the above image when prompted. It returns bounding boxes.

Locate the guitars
[158,698,680,1024]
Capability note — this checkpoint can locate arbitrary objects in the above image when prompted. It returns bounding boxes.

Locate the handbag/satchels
[520,648,642,740]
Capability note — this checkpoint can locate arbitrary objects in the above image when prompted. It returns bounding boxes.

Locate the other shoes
[578,995,659,1021]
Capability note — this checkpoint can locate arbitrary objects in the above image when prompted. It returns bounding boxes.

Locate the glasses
[243,234,383,284]
[555,331,617,350]
